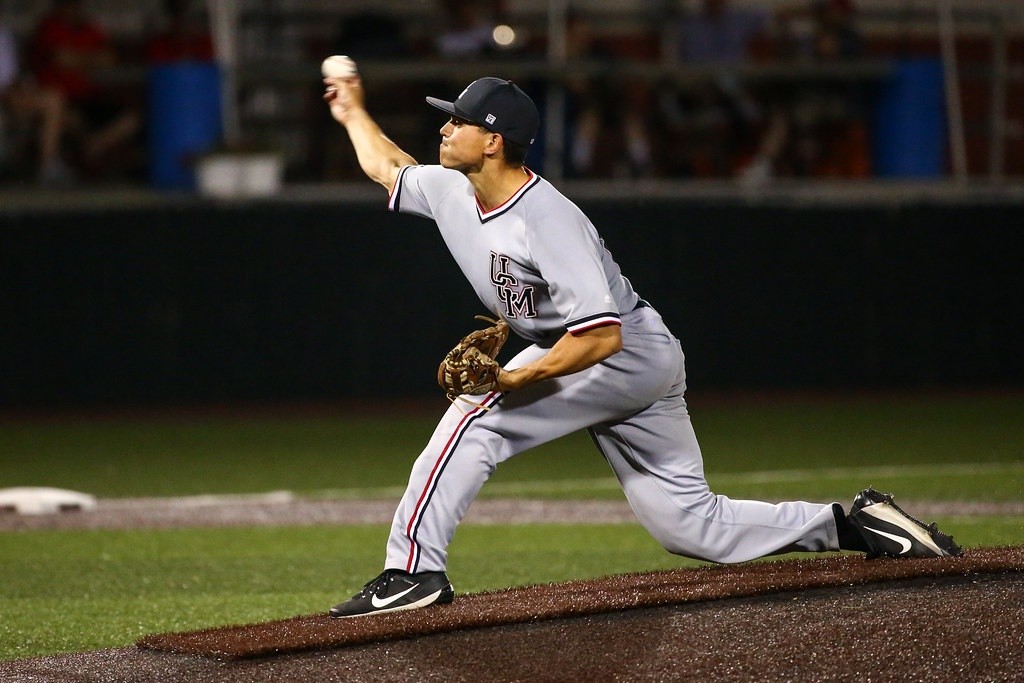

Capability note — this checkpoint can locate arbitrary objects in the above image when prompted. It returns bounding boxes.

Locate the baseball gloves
[436,320,510,396]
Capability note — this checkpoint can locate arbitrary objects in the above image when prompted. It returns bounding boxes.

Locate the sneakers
[328,569,454,619]
[845,481,964,559]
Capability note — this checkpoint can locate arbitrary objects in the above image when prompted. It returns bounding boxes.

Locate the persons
[323,76,963,617]
[435,0,876,180]
[0,0,213,180]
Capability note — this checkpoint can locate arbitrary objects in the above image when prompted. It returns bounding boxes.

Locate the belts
[631,300,650,311]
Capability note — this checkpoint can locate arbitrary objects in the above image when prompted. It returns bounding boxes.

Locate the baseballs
[322,54,358,81]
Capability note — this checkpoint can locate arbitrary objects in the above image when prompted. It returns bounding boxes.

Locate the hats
[426,76,539,147]
